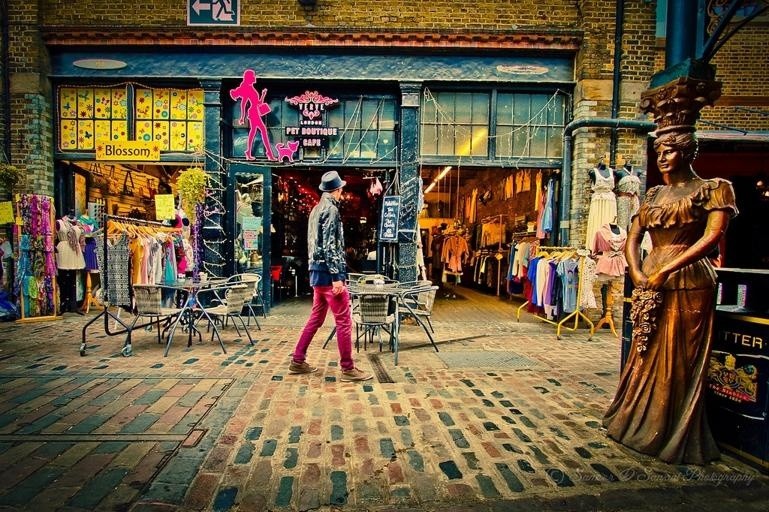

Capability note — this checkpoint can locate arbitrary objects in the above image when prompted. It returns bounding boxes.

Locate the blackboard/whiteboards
[379,196,400,241]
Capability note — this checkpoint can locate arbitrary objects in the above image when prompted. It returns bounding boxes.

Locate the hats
[319,171,347,192]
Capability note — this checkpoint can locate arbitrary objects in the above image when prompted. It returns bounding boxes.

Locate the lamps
[435,166,452,181]
[298,1,317,11]
[424,183,436,195]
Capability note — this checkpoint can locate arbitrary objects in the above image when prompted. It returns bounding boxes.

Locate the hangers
[536,246,576,259]
[92,216,177,238]
[489,217,506,224]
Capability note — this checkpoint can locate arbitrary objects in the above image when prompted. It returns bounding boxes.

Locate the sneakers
[341,367,373,382]
[289,360,317,373]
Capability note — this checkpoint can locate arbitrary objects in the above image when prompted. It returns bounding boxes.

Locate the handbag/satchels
[123,186,136,197]
[139,187,157,199]
[92,165,119,196]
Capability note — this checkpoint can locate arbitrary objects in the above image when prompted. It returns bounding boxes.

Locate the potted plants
[177,152,207,225]
[0,163,24,188]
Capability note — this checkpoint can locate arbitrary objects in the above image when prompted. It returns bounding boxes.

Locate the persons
[615,164,642,231]
[603,126,740,462]
[78,213,102,270]
[592,220,629,277]
[586,161,617,252]
[287,171,375,381]
[55,214,87,271]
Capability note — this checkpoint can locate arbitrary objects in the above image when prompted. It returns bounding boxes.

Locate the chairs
[124,272,263,358]
[346,274,440,367]
